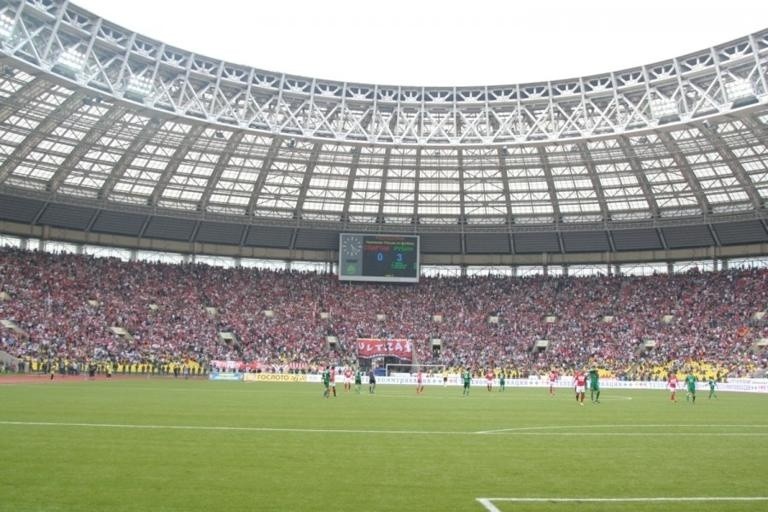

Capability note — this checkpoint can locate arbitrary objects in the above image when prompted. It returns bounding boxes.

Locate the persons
[585,365,600,403]
[342,364,351,389]
[321,365,331,399]
[546,368,560,396]
[416,368,423,393]
[441,368,450,387]
[665,371,680,402]
[484,366,495,391]
[572,368,588,407]
[353,367,363,393]
[497,366,506,392]
[707,373,719,400]
[330,367,337,396]
[683,369,698,402]
[0,244,767,378]
[368,365,376,393]
[460,366,472,397]
